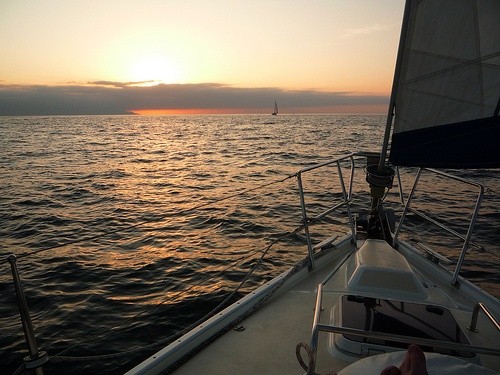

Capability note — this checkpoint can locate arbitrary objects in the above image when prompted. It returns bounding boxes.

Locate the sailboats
[272,99,279,115]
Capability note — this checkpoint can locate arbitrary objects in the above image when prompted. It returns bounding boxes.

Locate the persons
[398,342,428,375]
[380,365,404,375]
